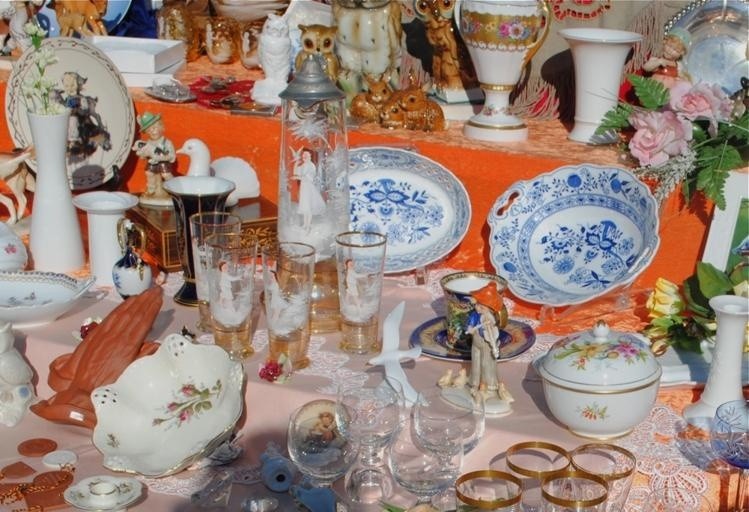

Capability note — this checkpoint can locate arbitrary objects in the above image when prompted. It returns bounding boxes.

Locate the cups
[338,231,387,353]
[162,177,236,307]
[188,212,242,334]
[539,471,610,512]
[441,271,509,349]
[206,234,259,357]
[455,470,523,512]
[571,443,636,512]
[506,441,570,512]
[259,242,315,369]
[91,486,124,507]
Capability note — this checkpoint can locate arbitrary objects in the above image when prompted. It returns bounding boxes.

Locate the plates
[488,161,664,309]
[664,1,749,92]
[0,271,97,325]
[64,474,144,510]
[3,36,136,191]
[412,316,534,363]
[90,334,245,478]
[349,147,471,274]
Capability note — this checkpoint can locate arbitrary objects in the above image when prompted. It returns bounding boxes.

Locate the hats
[470,282,504,312]
[138,111,160,133]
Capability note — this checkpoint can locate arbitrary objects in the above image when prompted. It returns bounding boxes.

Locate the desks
[0,47,749,512]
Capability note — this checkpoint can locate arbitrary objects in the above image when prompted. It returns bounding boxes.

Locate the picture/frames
[700,171,749,275]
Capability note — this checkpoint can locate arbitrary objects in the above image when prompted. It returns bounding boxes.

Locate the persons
[306,411,336,447]
[644,27,694,89]
[290,151,325,228]
[28,284,165,428]
[62,72,89,149]
[465,281,504,399]
[137,111,176,197]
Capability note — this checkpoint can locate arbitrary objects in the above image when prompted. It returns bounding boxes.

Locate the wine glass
[334,386,400,506]
[288,403,356,512]
[391,416,464,512]
[339,374,405,465]
[414,385,486,499]
[709,400,749,511]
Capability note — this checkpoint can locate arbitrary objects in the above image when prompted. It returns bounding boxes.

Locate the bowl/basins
[534,320,662,441]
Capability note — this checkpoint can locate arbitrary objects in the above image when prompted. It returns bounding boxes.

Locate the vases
[28,107,87,272]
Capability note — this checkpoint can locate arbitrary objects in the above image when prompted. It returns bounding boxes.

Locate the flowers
[589,63,749,209]
[644,264,748,350]
[22,22,72,115]
